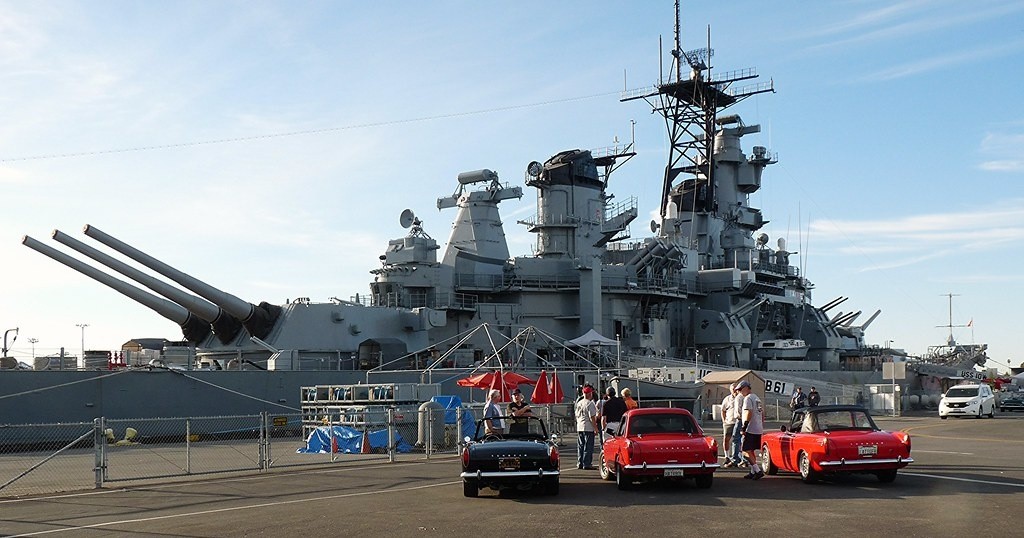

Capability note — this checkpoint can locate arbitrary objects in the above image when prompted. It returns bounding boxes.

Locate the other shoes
[582,466,598,470]
[724,458,731,464]
[737,462,748,468]
[744,471,765,479]
[724,462,738,468]
[577,465,582,468]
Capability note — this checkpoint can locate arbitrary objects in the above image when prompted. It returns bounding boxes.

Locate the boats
[607,376,706,399]
[752,338,811,360]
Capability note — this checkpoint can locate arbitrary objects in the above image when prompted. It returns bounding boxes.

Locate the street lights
[28,338,40,358]
[76,323,90,371]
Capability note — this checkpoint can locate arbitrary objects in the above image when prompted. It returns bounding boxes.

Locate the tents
[563,329,621,370]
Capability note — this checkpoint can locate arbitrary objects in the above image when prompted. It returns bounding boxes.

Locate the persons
[575,387,599,470]
[808,386,820,406]
[720,381,765,480]
[601,386,628,443]
[507,388,533,434]
[483,389,505,435]
[791,387,807,411]
[620,387,638,410]
[574,387,608,450]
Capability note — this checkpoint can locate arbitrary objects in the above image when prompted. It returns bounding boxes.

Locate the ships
[0,0,990,442]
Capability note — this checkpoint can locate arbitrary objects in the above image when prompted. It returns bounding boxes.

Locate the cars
[999,390,1024,412]
[760,404,914,485]
[938,384,996,419]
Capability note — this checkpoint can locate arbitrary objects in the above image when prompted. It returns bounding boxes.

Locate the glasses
[738,386,746,391]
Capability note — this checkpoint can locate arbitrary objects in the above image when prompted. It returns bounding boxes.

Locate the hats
[511,388,521,395]
[582,387,595,393]
[735,381,750,390]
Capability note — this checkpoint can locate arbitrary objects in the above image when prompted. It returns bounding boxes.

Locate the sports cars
[599,407,718,489]
[460,415,560,497]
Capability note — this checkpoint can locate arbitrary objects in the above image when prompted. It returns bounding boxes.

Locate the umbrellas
[457,371,563,404]
